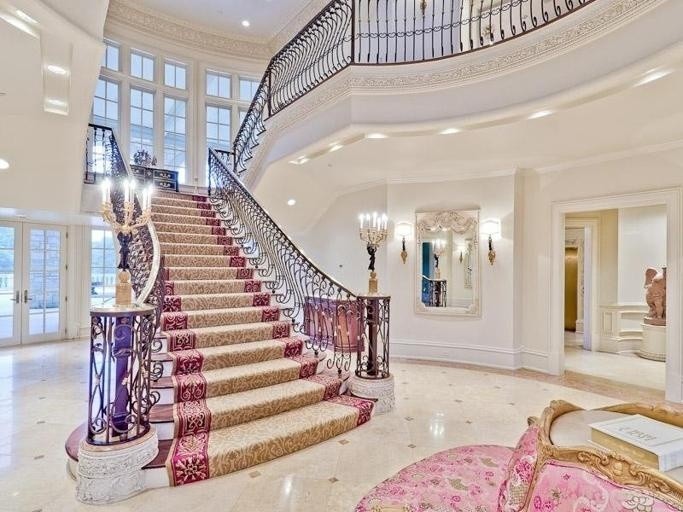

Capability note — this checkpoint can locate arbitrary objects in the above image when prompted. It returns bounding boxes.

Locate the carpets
[149,192,373,486]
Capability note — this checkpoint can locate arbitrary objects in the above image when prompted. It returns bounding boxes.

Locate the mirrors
[415,206,481,318]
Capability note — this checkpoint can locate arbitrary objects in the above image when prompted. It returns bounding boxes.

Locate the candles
[101,176,152,212]
[360,210,389,230]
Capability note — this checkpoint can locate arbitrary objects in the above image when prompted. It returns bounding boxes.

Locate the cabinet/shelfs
[130,166,178,191]
